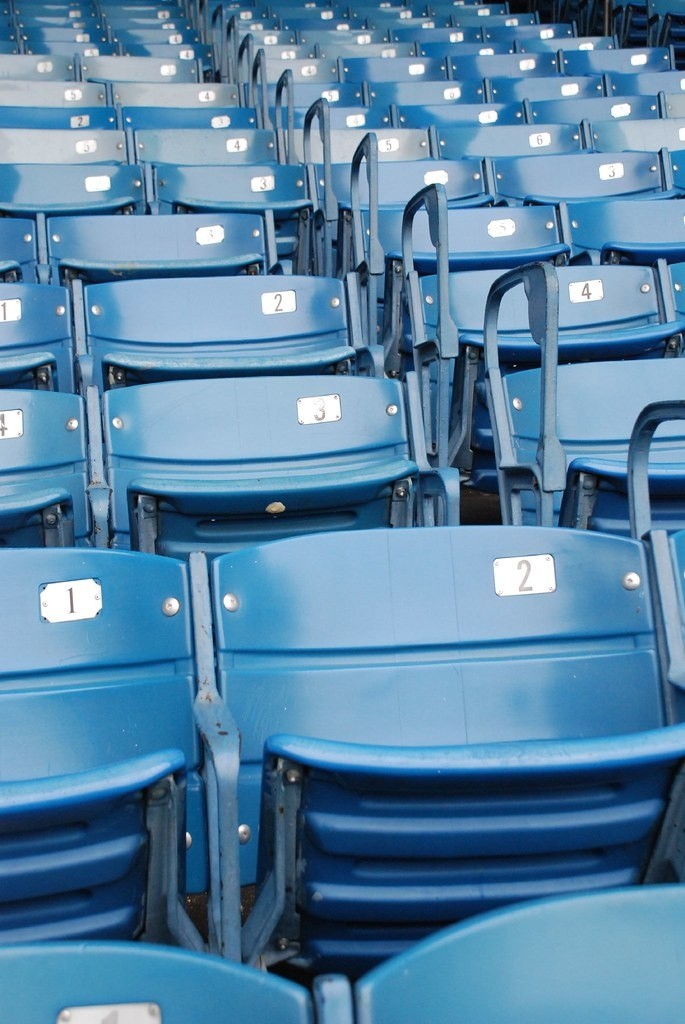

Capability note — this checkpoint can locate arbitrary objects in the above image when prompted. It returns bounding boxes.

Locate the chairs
[1,0,684,1024]
